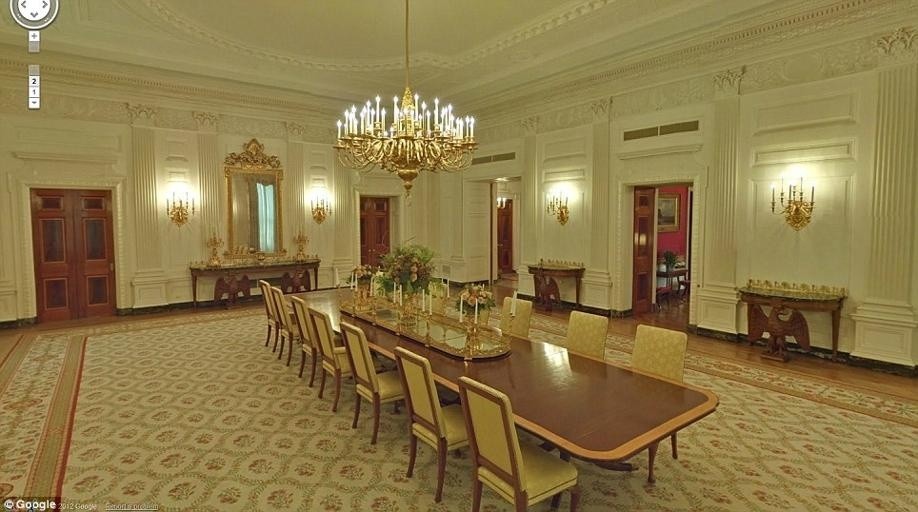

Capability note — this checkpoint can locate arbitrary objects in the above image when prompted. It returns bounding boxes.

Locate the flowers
[377,237,438,298]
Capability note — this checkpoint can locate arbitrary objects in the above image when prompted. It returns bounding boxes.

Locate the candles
[334,263,536,339]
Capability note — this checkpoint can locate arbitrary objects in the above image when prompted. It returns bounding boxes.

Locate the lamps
[329,0,481,202]
[767,172,818,231]
[165,189,196,228]
[291,223,312,262]
[204,222,224,268]
[309,195,333,226]
[544,192,571,227]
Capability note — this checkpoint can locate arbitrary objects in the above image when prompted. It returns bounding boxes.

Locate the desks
[737,284,845,362]
[188,258,321,312]
[525,264,585,313]
[655,267,688,300]
[277,283,722,510]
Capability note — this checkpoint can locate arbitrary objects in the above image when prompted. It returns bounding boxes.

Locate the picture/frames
[656,192,681,233]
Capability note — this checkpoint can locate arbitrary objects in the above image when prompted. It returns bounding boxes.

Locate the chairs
[251,279,405,446]
[629,322,691,483]
[392,343,471,503]
[564,307,609,363]
[452,373,584,512]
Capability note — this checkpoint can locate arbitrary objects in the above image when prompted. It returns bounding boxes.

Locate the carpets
[1,289,917,512]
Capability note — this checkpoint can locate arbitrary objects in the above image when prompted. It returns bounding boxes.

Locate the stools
[655,286,672,312]
[676,280,691,301]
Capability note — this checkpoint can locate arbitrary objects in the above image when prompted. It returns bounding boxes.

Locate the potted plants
[662,250,681,274]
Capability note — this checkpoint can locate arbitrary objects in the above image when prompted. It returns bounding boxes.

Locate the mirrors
[220,134,289,264]
